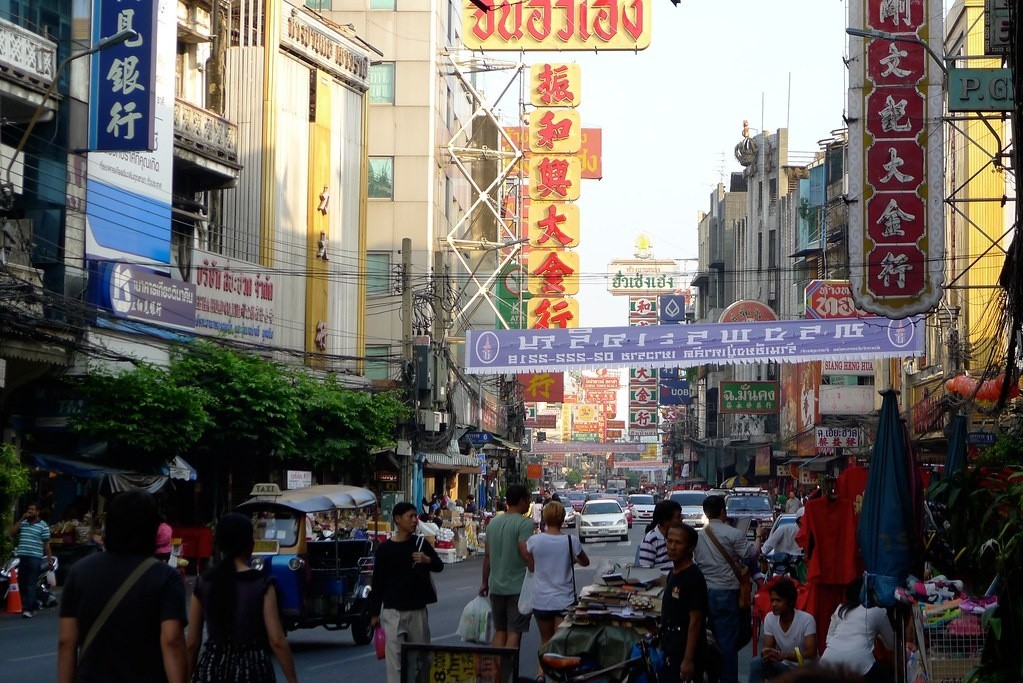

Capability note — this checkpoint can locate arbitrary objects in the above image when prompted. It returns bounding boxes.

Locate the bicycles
[517,624,680,683]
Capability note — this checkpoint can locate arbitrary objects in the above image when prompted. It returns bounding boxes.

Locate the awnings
[808,455,842,471]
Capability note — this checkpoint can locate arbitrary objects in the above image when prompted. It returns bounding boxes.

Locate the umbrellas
[720,475,749,488]
[943,410,968,474]
[857,385,923,683]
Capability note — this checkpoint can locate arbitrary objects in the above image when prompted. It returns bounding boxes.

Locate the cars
[541,496,576,528]
[565,493,589,514]
[723,487,782,542]
[627,494,656,521]
[597,497,633,528]
[770,513,798,536]
[577,499,629,545]
[663,490,711,532]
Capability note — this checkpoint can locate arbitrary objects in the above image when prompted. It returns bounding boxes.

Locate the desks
[44,543,98,585]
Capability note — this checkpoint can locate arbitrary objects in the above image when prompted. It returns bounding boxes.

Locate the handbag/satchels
[665,600,687,635]
[459,595,495,644]
[740,575,752,607]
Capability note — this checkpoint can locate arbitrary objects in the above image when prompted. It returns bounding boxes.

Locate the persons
[477,484,533,649]
[527,500,589,682]
[156,515,173,563]
[421,490,562,528]
[7,503,53,618]
[304,513,313,538]
[55,490,189,683]
[183,512,297,683]
[368,501,444,683]
[634,485,895,683]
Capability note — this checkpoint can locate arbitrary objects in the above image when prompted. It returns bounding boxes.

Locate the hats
[155,523,172,553]
[796,507,805,519]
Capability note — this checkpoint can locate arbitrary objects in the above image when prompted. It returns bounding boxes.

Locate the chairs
[730,499,766,508]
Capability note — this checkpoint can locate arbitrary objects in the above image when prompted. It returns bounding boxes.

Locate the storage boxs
[367,509,486,563]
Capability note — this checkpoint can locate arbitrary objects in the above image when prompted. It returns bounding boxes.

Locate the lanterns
[946,372,1020,403]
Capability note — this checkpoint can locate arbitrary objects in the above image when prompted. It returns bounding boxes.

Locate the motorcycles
[221,484,383,644]
[1,547,59,615]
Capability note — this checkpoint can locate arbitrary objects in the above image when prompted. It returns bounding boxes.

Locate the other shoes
[32,609,41,616]
[536,674,546,683]
[23,611,32,617]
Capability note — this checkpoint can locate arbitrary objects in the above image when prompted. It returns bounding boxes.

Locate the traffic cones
[4,567,23,615]
